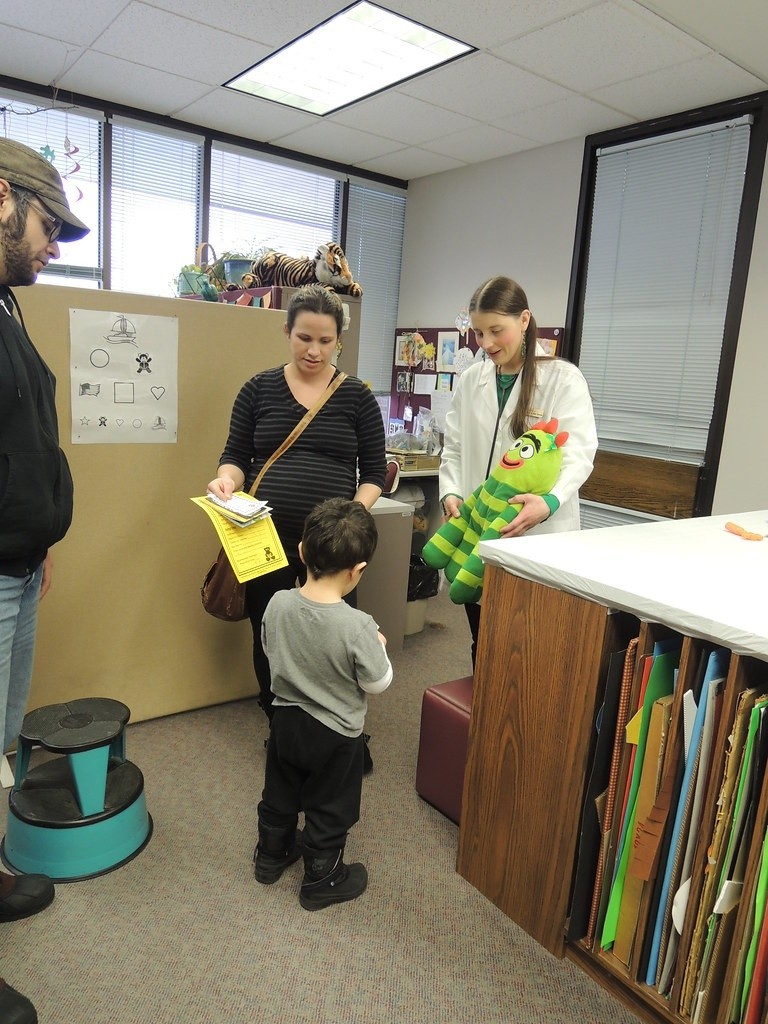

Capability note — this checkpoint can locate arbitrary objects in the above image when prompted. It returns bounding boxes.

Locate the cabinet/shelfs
[281,286,362,379]
[454,510,768,1024]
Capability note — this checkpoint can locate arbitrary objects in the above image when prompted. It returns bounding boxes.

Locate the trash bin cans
[404,554,433,636]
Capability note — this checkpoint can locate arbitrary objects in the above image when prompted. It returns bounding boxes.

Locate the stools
[0,697,154,884]
[415,675,473,829]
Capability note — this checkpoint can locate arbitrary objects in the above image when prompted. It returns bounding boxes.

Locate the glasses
[9,188,64,243]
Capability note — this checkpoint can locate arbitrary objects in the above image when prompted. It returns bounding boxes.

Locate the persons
[0,136,92,1024]
[438,277,600,677]
[207,286,389,774]
[253,497,393,910]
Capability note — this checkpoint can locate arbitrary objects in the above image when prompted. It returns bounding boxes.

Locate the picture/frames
[435,331,460,373]
[423,354,434,370]
[394,335,419,367]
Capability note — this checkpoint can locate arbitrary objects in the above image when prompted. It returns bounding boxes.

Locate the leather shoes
[0,873,56,923]
[0,983,38,1024]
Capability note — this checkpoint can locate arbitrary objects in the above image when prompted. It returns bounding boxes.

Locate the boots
[299,831,369,912]
[253,820,305,885]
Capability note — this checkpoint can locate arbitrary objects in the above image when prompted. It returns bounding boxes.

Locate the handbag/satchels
[199,544,257,620]
[407,553,441,600]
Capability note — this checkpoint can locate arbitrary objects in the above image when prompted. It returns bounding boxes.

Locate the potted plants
[223,237,257,289]
[177,263,210,295]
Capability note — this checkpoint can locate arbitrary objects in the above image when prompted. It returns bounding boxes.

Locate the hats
[0,137,91,243]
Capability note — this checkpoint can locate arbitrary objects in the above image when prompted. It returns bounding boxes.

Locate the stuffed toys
[226,241,363,296]
[422,418,569,605]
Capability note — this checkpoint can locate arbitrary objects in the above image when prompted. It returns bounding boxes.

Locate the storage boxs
[386,448,441,471]
